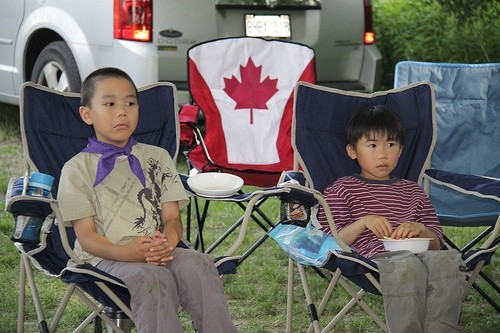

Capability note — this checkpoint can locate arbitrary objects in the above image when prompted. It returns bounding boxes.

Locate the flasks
[106,309,132,333]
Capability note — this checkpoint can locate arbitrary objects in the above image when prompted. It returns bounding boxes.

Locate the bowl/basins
[377,237,434,255]
[186,172,244,198]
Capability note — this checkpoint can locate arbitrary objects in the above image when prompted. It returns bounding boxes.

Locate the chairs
[5,36,500,333]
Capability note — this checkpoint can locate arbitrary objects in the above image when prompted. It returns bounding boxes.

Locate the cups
[17,171,55,242]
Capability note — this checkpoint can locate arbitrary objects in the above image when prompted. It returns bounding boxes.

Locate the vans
[0,0,382,130]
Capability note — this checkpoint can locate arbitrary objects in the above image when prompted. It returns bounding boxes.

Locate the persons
[56,68,237,333]
[318,106,465,333]
